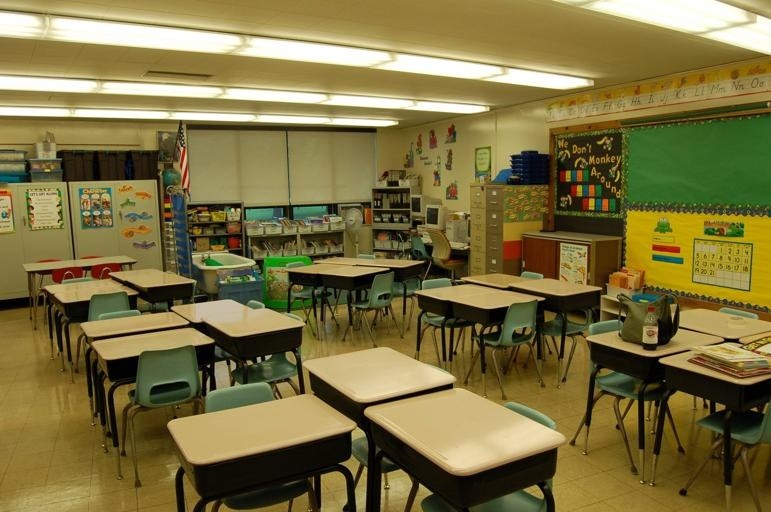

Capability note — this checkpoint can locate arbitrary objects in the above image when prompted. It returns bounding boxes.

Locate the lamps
[0,102,403,130]
[0,73,492,121]
[0,6,597,93]
[554,0,770,56]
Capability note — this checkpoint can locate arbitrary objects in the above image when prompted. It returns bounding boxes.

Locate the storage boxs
[0,161,27,173]
[0,148,26,163]
[94,149,130,180]
[28,169,63,182]
[37,141,57,158]
[192,252,264,301]
[58,147,96,182]
[607,284,644,300]
[127,148,162,180]
[27,157,63,169]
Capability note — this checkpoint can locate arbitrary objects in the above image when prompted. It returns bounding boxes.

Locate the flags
[176,124,191,198]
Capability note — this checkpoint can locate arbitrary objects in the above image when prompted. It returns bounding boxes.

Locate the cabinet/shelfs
[247,203,343,261]
[467,184,503,275]
[600,296,649,321]
[521,234,622,295]
[371,185,421,259]
[186,200,247,257]
[68,177,165,270]
[0,180,75,302]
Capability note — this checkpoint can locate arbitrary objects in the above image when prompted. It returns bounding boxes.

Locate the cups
[227,237,240,248]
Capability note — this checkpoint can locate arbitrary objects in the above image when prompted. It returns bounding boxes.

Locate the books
[608,267,645,290]
[689,340,771,378]
[245,215,344,258]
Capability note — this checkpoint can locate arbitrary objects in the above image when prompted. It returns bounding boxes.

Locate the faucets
[201,254,208,262]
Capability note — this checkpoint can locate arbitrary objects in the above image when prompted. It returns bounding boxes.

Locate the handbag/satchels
[616,291,681,347]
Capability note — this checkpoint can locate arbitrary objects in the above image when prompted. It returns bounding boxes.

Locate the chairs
[351,436,401,489]
[570,306,771,511]
[423,228,466,280]
[414,271,602,402]
[421,402,558,512]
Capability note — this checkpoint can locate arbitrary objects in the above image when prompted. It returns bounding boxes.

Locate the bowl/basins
[210,244,225,252]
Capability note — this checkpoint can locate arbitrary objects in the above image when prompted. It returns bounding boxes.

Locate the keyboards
[447,241,469,249]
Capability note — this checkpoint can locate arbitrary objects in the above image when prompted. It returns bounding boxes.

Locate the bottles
[641,306,659,351]
[226,207,241,214]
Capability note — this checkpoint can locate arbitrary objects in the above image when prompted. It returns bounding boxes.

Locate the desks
[286,257,428,348]
[73,298,306,488]
[425,240,467,251]
[166,394,356,511]
[363,388,568,512]
[22,255,198,385]
[303,348,457,510]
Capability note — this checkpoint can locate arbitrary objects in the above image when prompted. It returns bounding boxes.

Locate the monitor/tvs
[411,195,441,217]
[425,204,455,230]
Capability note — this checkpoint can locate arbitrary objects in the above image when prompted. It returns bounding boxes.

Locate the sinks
[191,253,249,267]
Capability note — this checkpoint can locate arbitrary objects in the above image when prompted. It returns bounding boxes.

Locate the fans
[342,207,365,255]
